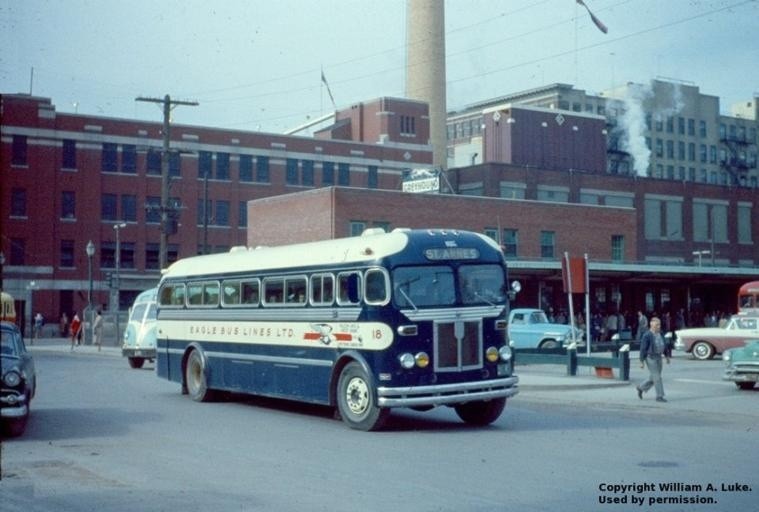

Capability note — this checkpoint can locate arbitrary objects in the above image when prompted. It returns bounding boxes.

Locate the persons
[69,311,82,352]
[59,311,70,338]
[92,307,104,352]
[545,303,736,343]
[634,317,672,403]
[33,312,46,338]
[161,279,350,302]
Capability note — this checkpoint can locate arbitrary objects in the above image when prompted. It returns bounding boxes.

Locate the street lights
[80,237,99,305]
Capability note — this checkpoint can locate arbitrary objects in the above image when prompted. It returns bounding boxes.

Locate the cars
[508,307,581,351]
[664,311,759,390]
[0,320,37,439]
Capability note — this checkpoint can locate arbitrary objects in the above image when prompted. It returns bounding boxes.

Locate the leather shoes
[636,386,643,400]
[656,397,667,402]
